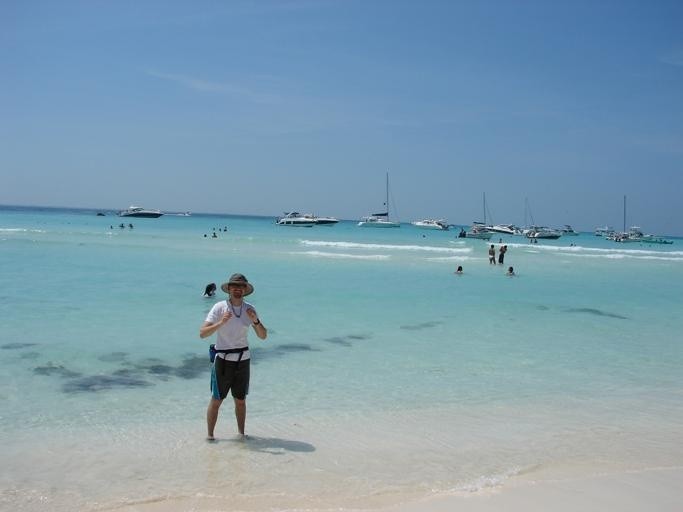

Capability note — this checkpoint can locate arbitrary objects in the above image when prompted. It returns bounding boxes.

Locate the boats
[276,211,319,225]
[460,223,495,240]
[642,239,673,244]
[526,225,561,240]
[592,223,616,236]
[611,225,654,242]
[164,211,191,216]
[316,217,339,224]
[120,206,163,219]
[486,223,530,235]
[560,225,579,236]
[411,219,448,231]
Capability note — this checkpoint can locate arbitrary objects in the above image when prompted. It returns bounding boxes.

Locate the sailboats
[358,168,401,228]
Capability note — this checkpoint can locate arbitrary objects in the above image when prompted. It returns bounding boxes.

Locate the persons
[203,226,228,239]
[129,224,132,229]
[499,245,508,265]
[535,238,538,243]
[110,225,113,229]
[201,271,268,441]
[574,242,577,246]
[120,223,125,228]
[489,244,497,264]
[210,283,217,296]
[608,234,620,242]
[455,266,465,275]
[498,238,503,243]
[530,239,534,244]
[422,234,426,238]
[506,267,515,275]
[201,284,213,297]
[571,244,574,246]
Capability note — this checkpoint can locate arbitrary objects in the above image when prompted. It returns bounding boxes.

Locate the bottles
[208,344,215,362]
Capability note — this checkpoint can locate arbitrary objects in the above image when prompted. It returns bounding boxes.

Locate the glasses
[229,283,246,289]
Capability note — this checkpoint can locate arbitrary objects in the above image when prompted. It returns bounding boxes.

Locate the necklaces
[229,298,243,318]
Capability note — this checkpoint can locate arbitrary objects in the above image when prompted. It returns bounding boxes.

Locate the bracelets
[253,319,260,325]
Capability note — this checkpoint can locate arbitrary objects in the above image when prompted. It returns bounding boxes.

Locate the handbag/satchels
[209,344,216,363]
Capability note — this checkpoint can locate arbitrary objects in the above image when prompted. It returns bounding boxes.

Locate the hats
[221,273,253,297]
